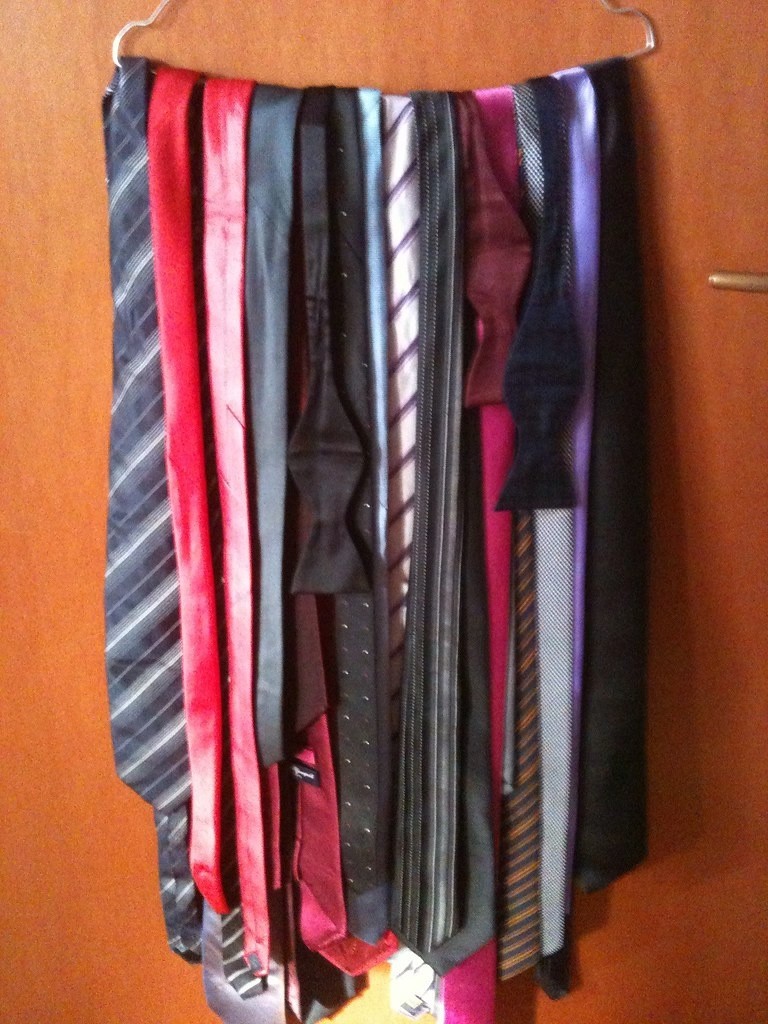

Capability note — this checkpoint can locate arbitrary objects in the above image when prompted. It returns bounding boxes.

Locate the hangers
[110,2,657,91]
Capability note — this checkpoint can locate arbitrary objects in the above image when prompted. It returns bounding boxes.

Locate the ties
[98,52,653,1024]
